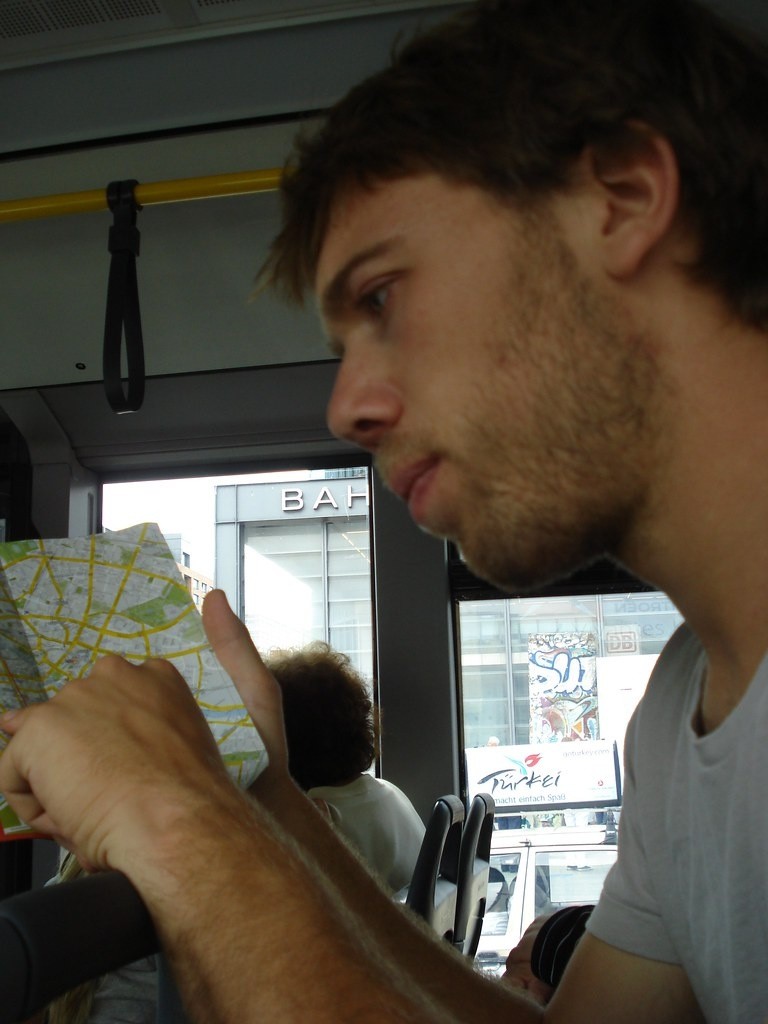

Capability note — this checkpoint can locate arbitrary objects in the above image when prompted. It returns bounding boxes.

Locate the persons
[44,842,160,1024]
[497,904,596,1008]
[0,0,768,1024]
[486,736,500,746]
[267,644,425,895]
[498,810,594,873]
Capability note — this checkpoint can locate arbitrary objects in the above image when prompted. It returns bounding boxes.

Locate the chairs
[404,793,496,960]
[0,869,184,1024]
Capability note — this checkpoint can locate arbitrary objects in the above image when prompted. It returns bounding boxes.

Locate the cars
[467,825,618,980]
